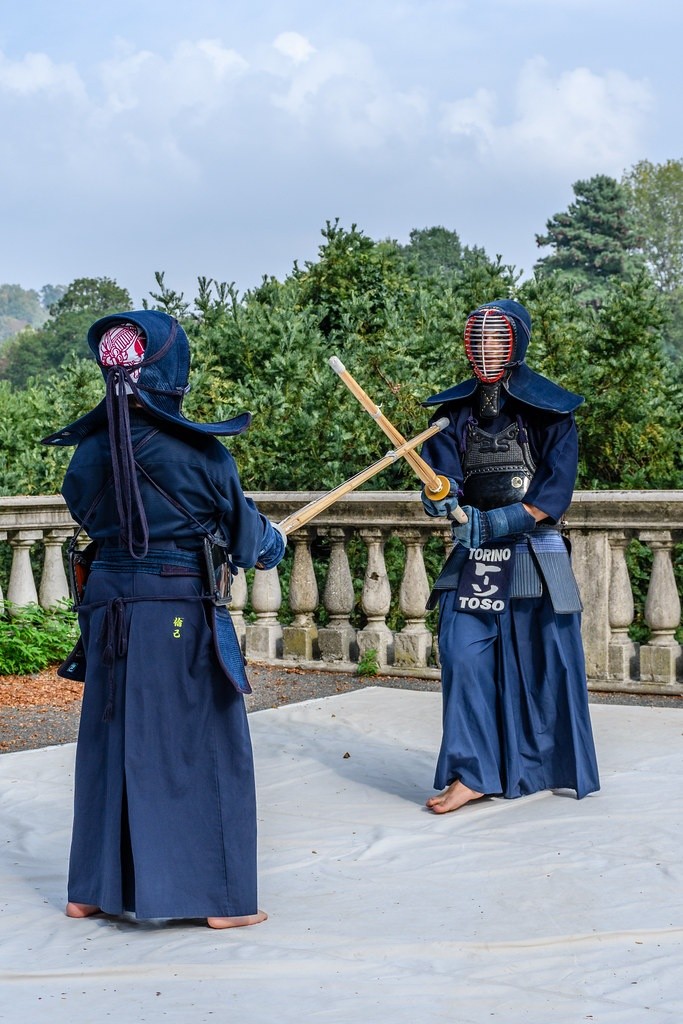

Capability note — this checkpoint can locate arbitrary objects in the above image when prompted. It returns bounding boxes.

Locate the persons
[420,299,600,814]
[40,309,288,931]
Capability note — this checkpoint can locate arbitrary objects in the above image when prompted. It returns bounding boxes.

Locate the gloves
[420,476,535,548]
[254,511,289,570]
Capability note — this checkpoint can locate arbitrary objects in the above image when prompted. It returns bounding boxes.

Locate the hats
[38,309,251,446]
[421,299,585,418]
